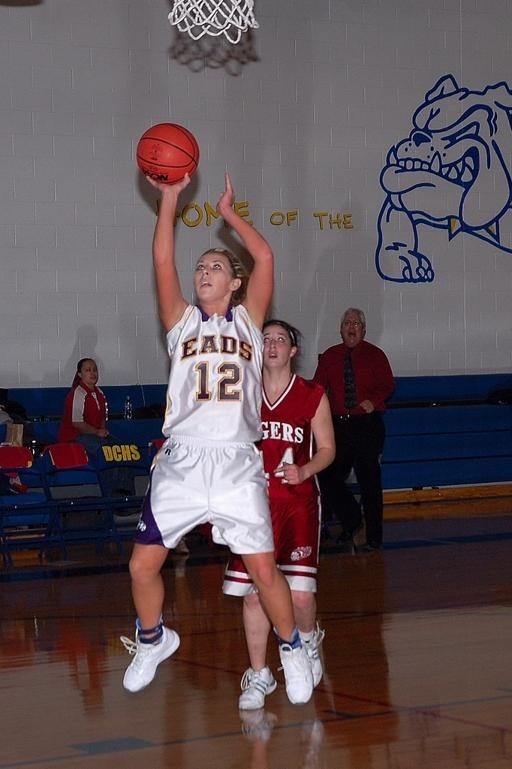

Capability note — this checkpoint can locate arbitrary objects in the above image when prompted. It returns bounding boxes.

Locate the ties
[341,346,358,409]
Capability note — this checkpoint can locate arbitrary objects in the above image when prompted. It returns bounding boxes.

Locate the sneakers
[236,625,326,713]
[119,625,180,692]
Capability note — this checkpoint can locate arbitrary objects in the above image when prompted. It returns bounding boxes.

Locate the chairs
[1,411,169,570]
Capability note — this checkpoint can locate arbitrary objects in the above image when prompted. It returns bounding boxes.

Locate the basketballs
[136,123,199,183]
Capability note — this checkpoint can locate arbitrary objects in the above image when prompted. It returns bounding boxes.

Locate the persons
[119,175,318,706]
[52,607,108,716]
[58,359,130,496]
[237,704,328,769]
[239,316,342,709]
[309,309,396,555]
